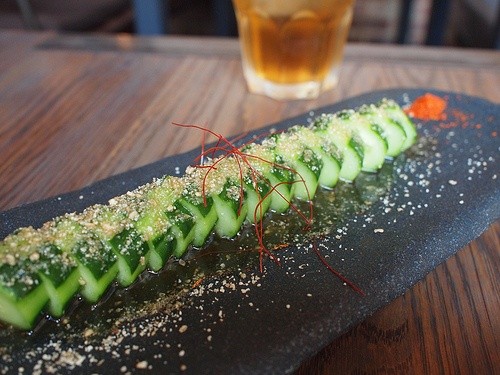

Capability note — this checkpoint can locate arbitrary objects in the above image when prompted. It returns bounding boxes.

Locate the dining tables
[0,27,500,375]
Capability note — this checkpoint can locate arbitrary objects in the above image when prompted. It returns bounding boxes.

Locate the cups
[231,0,351,99]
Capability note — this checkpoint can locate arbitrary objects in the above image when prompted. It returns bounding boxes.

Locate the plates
[0,85,500,373]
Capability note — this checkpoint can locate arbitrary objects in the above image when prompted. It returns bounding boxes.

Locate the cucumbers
[0,99,420,330]
